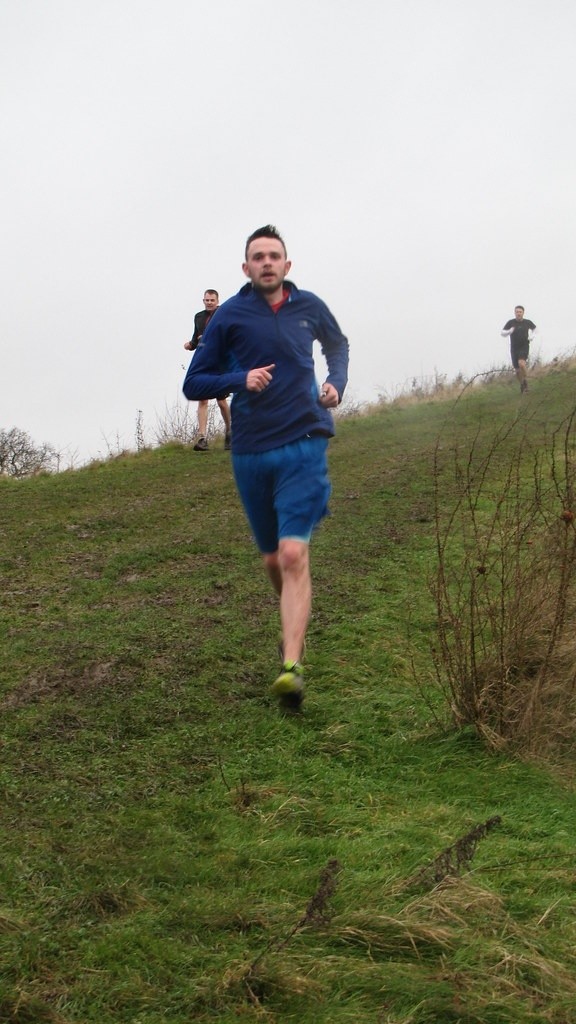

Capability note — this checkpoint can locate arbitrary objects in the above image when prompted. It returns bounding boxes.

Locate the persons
[503,306,536,393]
[185,226,349,705]
[184,289,231,450]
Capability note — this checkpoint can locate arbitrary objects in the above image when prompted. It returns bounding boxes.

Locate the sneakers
[273,661,306,692]
[194,438,209,450]
[225,435,231,449]
